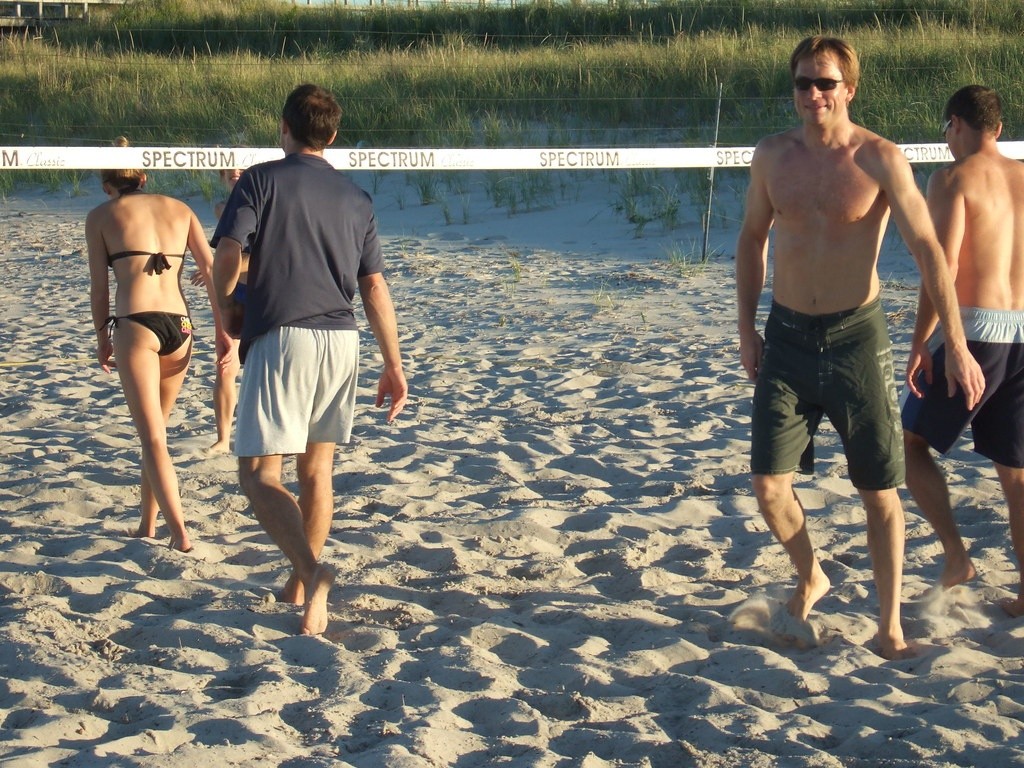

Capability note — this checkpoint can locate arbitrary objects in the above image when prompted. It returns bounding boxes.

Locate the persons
[898,84,1024,616]
[85,136,235,551]
[190,144,251,455]
[738,35,986,656]
[211,85,408,637]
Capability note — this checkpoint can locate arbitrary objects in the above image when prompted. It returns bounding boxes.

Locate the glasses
[795,76,845,91]
